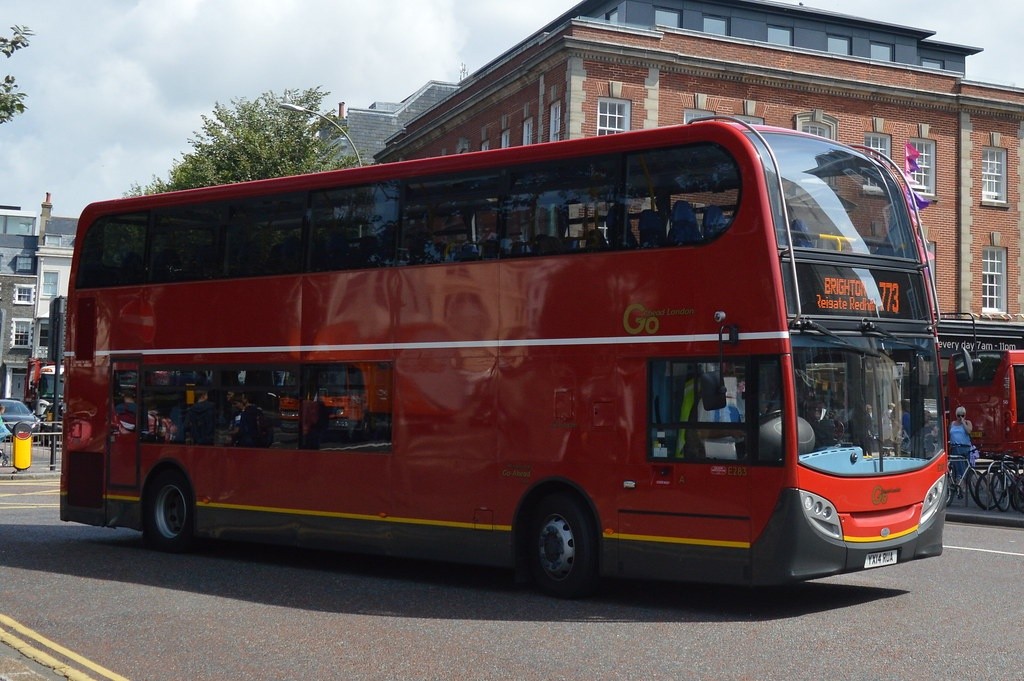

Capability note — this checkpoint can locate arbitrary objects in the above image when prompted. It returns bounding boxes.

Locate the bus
[64,114,1006,598]
[946,348,1023,463]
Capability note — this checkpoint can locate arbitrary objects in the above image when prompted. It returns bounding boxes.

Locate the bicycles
[942,441,1024,512]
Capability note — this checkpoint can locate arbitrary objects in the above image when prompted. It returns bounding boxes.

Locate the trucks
[280,365,395,441]
[22,359,64,445]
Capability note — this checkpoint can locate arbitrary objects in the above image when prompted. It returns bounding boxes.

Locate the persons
[828,398,939,459]
[173,385,275,447]
[948,406,973,486]
[0,403,13,444]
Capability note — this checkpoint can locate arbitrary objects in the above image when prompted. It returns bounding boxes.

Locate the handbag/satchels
[972,444,980,459]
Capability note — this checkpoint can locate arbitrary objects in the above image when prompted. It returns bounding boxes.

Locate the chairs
[118,199,818,286]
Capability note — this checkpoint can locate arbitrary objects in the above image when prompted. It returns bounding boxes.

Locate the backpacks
[250,410,274,448]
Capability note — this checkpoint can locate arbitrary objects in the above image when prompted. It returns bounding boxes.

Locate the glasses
[957,415,965,418]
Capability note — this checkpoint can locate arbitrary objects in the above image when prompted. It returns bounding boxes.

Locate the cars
[0,399,41,439]
